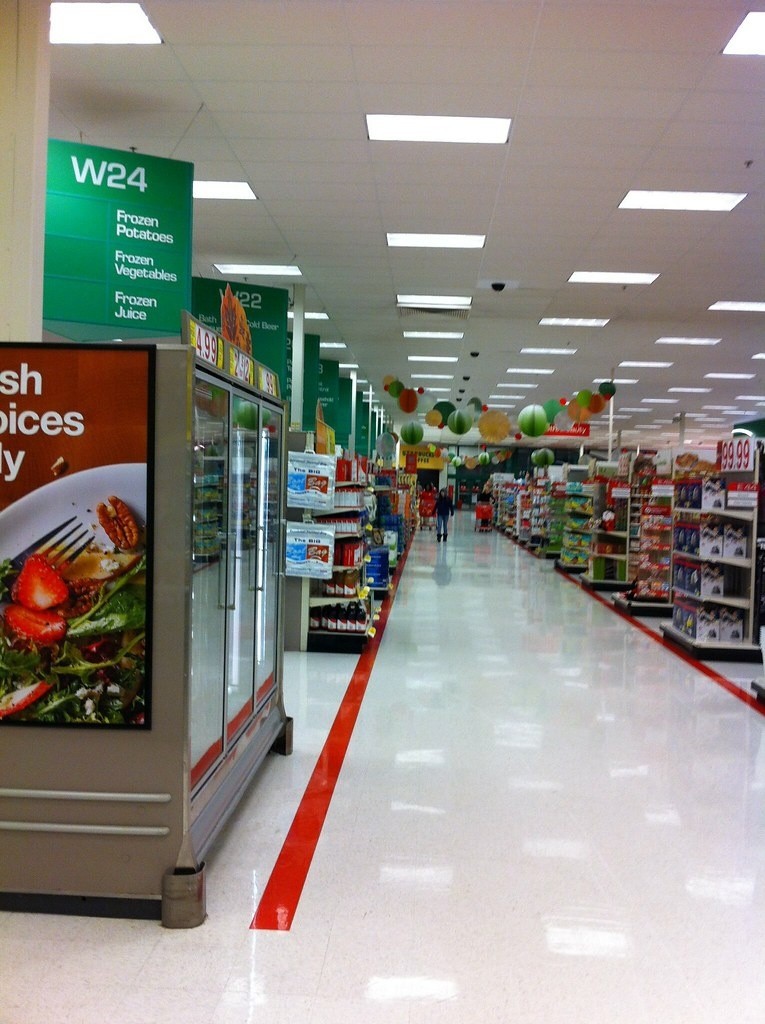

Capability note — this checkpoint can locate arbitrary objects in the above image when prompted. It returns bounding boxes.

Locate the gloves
[432,511,435,514]
[451,512,454,516]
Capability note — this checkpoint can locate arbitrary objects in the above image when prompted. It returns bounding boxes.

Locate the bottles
[309,570,370,633]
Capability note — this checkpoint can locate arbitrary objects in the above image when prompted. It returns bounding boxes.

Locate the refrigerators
[89,341,287,867]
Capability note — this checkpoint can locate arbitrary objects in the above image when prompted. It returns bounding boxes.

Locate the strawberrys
[6,605,66,642]
[18,554,68,610]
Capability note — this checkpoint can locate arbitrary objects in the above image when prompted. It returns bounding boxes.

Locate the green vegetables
[0,554,145,724]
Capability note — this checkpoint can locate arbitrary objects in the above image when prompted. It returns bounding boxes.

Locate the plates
[1,462,146,560]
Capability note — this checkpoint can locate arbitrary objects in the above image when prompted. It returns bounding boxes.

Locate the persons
[417,480,437,524]
[432,488,454,542]
[477,486,496,532]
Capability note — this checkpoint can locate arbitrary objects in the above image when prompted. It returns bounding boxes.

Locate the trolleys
[472,500,494,534]
[417,500,438,531]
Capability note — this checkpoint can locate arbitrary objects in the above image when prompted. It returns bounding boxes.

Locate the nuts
[96,495,139,550]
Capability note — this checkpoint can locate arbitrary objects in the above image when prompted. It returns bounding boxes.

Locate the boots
[443,533,448,541]
[437,533,442,542]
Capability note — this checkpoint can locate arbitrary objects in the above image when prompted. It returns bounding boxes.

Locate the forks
[2,517,94,571]
